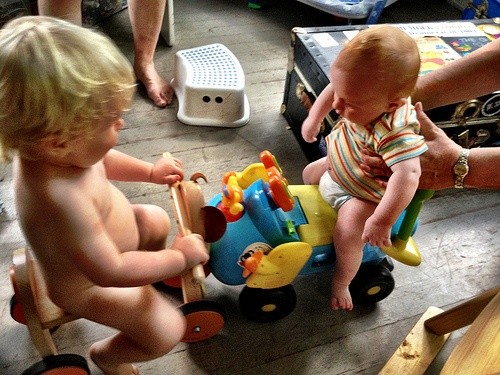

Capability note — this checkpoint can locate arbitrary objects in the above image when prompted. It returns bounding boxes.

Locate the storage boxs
[280,18,500,162]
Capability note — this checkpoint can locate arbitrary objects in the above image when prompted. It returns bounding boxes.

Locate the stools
[169,43,252,127]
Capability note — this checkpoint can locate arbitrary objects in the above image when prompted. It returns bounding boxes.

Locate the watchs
[454,147,471,190]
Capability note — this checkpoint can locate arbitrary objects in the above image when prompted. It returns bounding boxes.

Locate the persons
[360,36,500,192]
[38,0,175,107]
[0,15,211,375]
[300,24,430,314]
